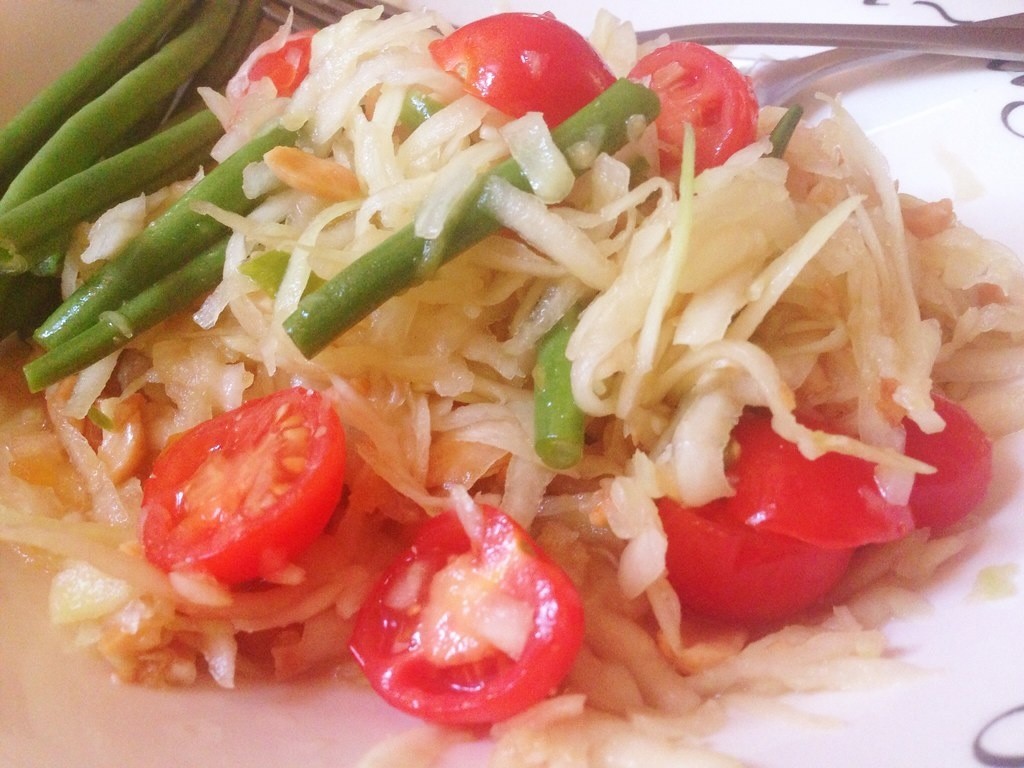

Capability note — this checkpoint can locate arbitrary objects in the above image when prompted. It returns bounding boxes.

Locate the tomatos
[346,507,586,727]
[659,392,993,628]
[429,17,760,174]
[138,388,343,582]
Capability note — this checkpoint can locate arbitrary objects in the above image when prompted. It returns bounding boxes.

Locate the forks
[257,1,1023,70]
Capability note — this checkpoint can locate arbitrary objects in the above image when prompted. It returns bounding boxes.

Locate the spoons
[738,13,1023,109]
